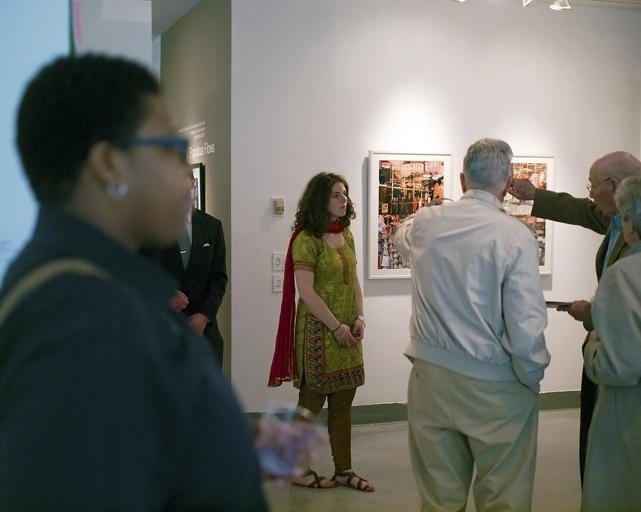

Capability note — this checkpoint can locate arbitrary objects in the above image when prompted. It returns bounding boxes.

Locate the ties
[177,222,192,269]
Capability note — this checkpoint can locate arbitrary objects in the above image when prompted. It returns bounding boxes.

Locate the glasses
[129,132,188,160]
[586,176,623,193]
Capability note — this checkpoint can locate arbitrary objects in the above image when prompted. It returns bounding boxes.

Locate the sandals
[334,468,376,492]
[295,464,336,489]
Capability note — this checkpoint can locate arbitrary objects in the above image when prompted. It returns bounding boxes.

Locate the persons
[266,171,376,493]
[507,150,641,499]
[580,175,641,512]
[392,138,552,512]
[0,53,272,512]
[157,166,230,375]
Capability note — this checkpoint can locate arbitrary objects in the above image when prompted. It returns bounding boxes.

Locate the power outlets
[272,273,284,292]
[272,251,285,271]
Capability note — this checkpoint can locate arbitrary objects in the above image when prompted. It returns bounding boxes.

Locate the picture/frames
[367,149,455,282]
[189,163,205,214]
[500,158,554,278]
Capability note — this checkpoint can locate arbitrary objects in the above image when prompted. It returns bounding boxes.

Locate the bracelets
[334,326,347,339]
[332,323,341,333]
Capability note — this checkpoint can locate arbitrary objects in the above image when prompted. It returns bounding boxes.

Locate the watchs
[356,315,366,327]
